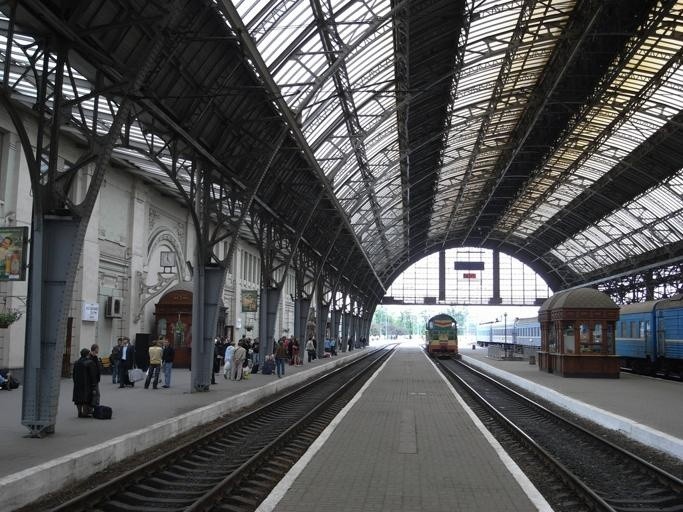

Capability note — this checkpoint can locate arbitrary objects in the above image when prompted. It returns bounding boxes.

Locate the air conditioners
[104,296,122,319]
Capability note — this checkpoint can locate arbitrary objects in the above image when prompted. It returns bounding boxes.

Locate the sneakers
[113,380,134,388]
[144,385,158,390]
[78,412,91,419]
[162,384,169,389]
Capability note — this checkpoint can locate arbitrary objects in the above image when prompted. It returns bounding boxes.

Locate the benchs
[99,357,113,375]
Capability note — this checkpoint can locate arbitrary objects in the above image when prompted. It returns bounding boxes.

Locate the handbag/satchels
[93,406,112,420]
[127,363,144,382]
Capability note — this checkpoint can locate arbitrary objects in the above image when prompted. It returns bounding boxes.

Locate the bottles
[11,250,20,275]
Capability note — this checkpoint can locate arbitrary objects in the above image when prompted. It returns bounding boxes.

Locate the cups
[5,254,12,275]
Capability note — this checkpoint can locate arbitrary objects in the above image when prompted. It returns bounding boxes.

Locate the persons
[87,344,99,414]
[359,335,363,349]
[0,236,12,262]
[210,334,354,385]
[108,337,136,388]
[0,368,19,391]
[143,337,175,389]
[362,337,366,348]
[71,348,99,418]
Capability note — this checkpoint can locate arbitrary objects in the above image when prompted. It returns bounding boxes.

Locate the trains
[474,289,683,385]
[421,313,463,361]
